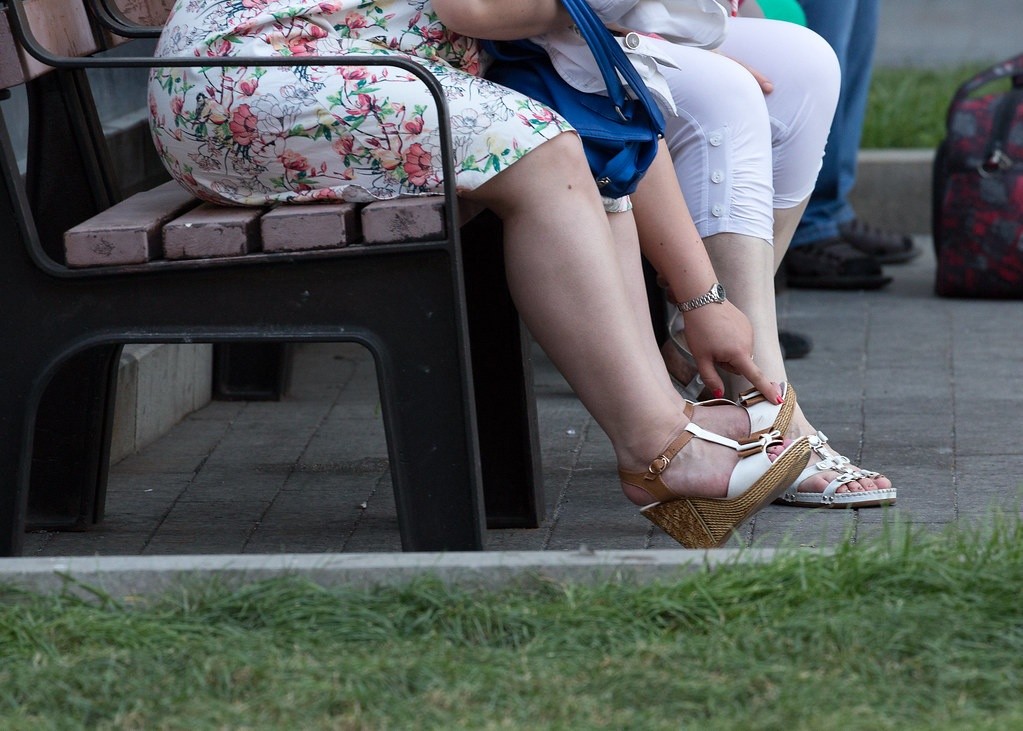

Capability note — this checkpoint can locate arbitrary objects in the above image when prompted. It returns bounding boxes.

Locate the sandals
[785,239,893,287]
[658,309,709,403]
[771,430,898,508]
[839,218,921,261]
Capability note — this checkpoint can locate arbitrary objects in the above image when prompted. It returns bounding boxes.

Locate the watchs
[676,283,726,313]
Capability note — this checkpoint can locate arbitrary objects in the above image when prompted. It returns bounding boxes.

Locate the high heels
[684,381,797,438]
[616,422,812,550]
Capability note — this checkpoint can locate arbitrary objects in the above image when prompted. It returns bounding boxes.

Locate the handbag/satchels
[929,53,1023,301]
[484,1,666,200]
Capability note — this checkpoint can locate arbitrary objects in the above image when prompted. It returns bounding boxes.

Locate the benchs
[0,0,547,556]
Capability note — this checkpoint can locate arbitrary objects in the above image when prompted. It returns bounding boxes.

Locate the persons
[603,0,896,507]
[148,0,811,549]
[783,0,921,292]
